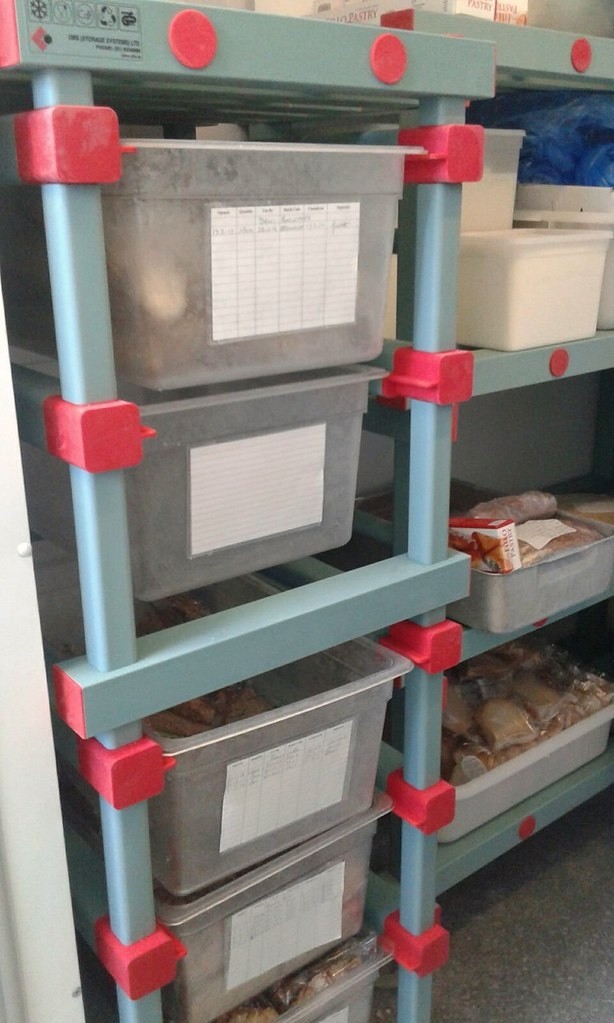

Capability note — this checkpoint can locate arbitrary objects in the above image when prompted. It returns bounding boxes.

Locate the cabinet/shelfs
[273,13,614,989]
[1,0,495,1023]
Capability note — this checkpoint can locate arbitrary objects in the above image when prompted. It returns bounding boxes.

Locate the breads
[139,490,614,1023]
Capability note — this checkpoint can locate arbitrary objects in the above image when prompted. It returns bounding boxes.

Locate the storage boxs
[354,477,614,636]
[376,663,614,843]
[458,227,614,351]
[13,363,391,600]
[28,540,414,898]
[460,128,527,230]
[213,928,397,1023]
[62,786,396,1023]
[516,181,614,330]
[254,1,614,39]
[0,137,427,391]
